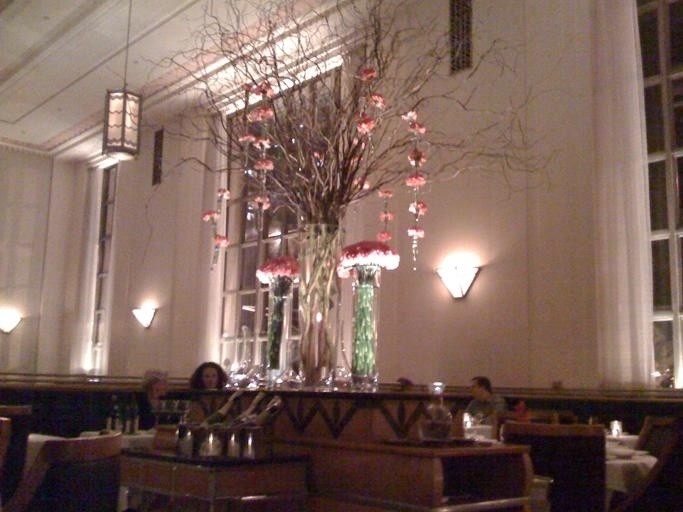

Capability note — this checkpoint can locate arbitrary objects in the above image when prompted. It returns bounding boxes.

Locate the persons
[463,376,508,436]
[136,368,170,430]
[190,362,228,389]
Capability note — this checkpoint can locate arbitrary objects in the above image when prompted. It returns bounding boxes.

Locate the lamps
[130,308,156,328]
[101,0,142,161]
[0,316,23,334]
[433,267,481,299]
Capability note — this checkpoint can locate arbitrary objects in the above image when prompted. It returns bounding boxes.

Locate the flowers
[336,241,400,286]
[255,257,303,295]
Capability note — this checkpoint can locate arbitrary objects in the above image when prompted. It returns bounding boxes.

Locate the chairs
[0,405,683,512]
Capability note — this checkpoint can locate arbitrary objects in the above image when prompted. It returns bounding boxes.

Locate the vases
[294,223,341,391]
[264,279,291,389]
[349,266,378,392]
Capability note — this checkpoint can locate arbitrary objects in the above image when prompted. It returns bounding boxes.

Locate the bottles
[105,394,142,437]
[420,381,452,442]
[193,388,284,432]
